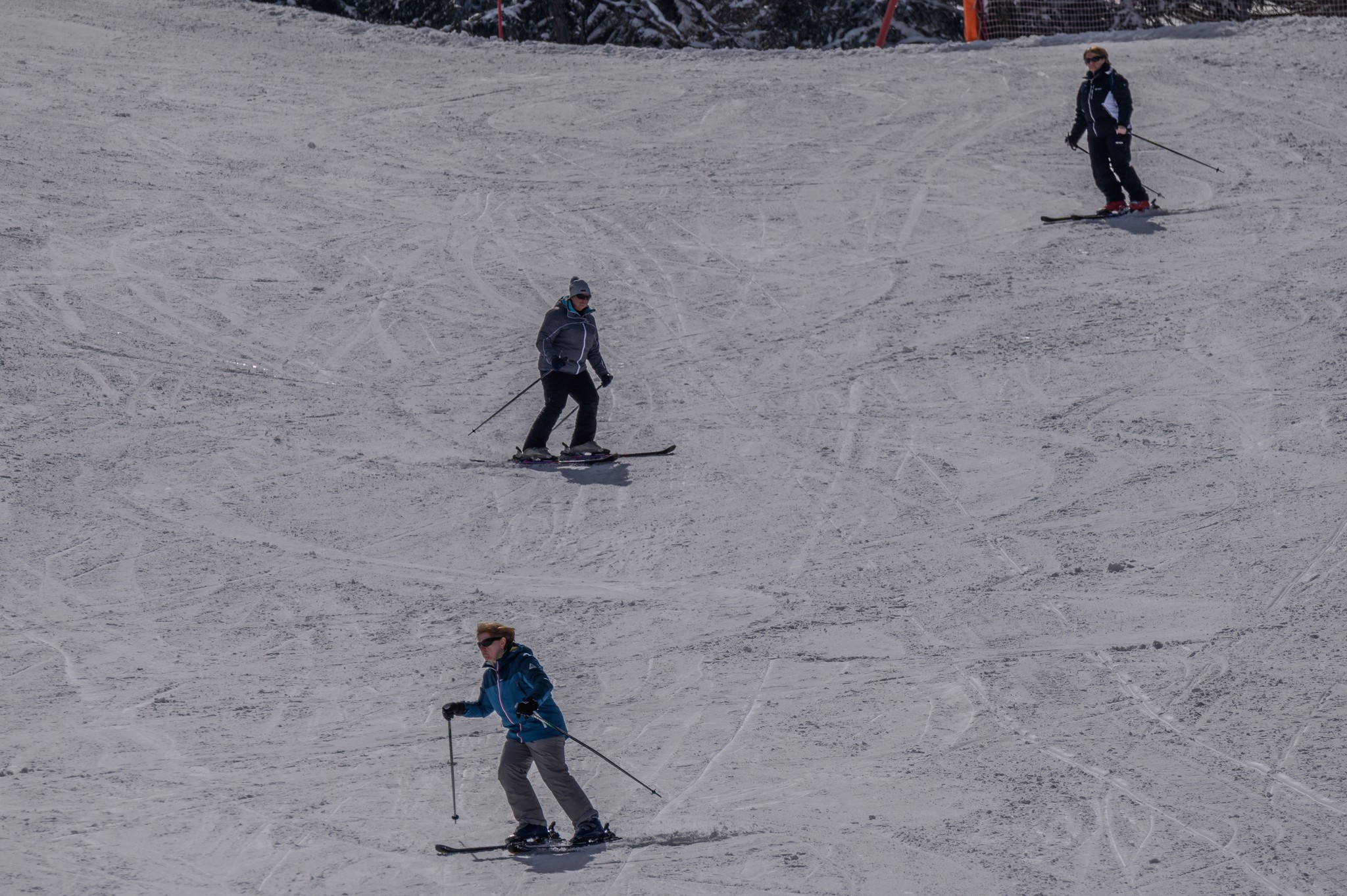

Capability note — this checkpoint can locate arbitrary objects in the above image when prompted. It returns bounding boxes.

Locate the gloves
[440,699,464,721]
[548,357,566,372]
[600,372,613,390]
[514,694,542,720]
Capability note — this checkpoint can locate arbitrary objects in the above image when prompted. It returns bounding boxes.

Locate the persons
[1068,47,1151,215]
[524,276,612,459]
[443,622,604,845]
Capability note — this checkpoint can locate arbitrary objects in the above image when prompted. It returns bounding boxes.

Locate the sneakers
[1123,198,1150,214]
[514,444,554,462]
[563,440,610,455]
[504,822,549,846]
[1096,199,1129,217]
[567,815,606,842]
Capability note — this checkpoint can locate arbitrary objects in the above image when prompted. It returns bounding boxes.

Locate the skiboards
[468,443,678,464]
[436,827,624,855]
[1041,197,1160,222]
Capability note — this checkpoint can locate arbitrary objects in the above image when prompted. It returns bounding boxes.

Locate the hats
[569,276,593,297]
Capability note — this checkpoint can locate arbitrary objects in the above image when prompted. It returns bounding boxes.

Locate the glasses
[575,293,592,302]
[475,636,504,650]
[1082,56,1110,65]
